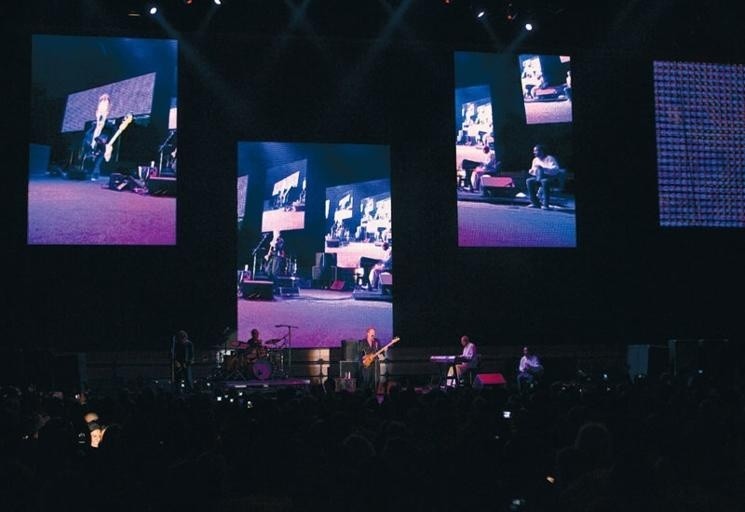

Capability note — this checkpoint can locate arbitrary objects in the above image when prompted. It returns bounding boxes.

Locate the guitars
[173,354,208,376]
[362,337,401,368]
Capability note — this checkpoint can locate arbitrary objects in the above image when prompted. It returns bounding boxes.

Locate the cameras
[497,406,512,422]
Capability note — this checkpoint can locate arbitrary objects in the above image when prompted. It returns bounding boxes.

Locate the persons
[441,334,478,389]
[515,345,544,387]
[245,329,261,348]
[264,233,286,294]
[362,242,393,290]
[525,143,562,210]
[79,92,119,177]
[175,329,196,386]
[471,145,498,190]
[359,328,385,362]
[1,378,744,512]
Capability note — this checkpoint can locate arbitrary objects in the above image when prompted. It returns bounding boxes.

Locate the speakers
[149,178,177,198]
[473,373,507,387]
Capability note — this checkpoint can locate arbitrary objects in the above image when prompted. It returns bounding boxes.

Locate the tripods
[214,345,288,382]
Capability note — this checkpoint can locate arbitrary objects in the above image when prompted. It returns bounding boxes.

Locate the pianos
[430,353,467,366]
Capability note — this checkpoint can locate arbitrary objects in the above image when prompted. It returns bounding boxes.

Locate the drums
[246,352,259,364]
[271,349,282,362]
[253,356,273,381]
[258,346,269,357]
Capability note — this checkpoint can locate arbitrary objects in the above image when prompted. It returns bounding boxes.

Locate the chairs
[537,173,564,205]
[378,271,393,294]
[464,353,482,385]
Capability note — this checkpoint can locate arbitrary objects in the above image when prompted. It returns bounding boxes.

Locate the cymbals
[237,340,245,344]
[265,338,281,343]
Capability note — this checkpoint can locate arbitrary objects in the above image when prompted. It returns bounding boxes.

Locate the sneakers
[529,204,550,210]
[362,284,374,289]
[465,186,476,190]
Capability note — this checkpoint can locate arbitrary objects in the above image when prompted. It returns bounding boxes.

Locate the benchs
[535,87,556,101]
[148,176,177,195]
